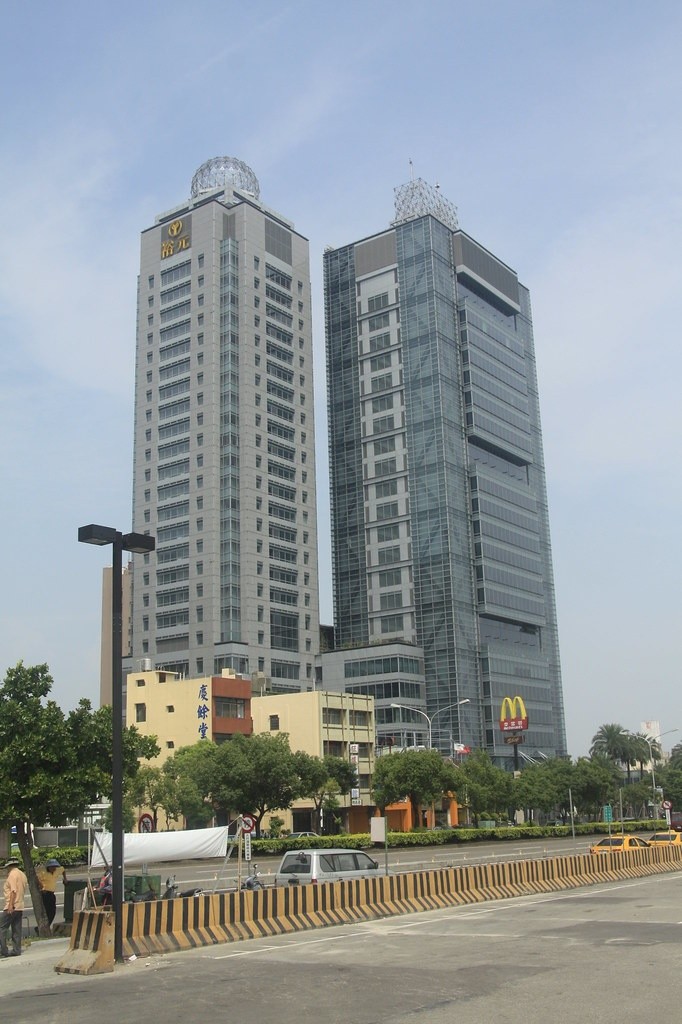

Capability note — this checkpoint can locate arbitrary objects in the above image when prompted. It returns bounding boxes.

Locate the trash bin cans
[63,873,161,922]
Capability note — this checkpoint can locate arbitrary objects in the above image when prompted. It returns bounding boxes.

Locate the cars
[590,833,653,855]
[10,842,61,850]
[646,829,682,848]
[285,831,320,839]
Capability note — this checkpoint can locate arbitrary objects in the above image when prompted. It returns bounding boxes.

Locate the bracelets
[94,887,98,892]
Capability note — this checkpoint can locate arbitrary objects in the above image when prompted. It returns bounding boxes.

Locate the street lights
[619,728,680,820]
[78,523,156,966]
[390,698,470,751]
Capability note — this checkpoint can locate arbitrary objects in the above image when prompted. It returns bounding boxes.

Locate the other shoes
[8,951,21,956]
[0,952,8,957]
[34,927,39,936]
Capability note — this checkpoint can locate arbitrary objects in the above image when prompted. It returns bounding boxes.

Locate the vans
[670,811,682,831]
[274,849,397,888]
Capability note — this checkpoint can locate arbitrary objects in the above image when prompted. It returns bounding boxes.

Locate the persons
[34,859,68,936]
[0,856,27,957]
[93,866,113,906]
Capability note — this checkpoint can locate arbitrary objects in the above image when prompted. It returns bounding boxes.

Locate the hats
[45,859,61,866]
[5,856,20,866]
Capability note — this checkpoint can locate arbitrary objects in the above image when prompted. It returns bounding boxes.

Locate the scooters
[161,874,205,900]
[235,862,267,891]
[95,865,159,907]
